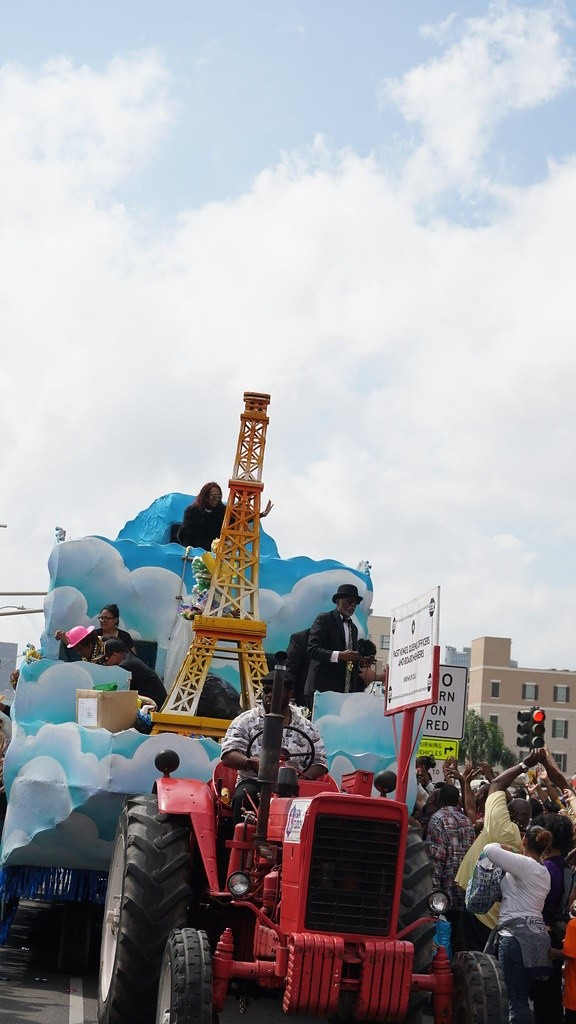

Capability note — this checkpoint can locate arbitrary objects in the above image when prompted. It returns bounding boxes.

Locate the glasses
[98,617,114,622]
[210,494,222,500]
[263,686,273,694]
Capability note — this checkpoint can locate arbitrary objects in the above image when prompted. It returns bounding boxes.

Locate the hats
[92,639,127,660]
[332,584,363,605]
[65,625,95,648]
[260,671,295,686]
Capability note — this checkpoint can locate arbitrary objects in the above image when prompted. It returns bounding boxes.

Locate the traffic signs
[416,737,460,762]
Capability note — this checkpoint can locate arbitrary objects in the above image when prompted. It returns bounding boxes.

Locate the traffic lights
[516,710,532,747]
[529,708,546,748]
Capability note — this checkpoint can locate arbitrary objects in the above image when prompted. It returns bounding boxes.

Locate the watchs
[519,762,529,773]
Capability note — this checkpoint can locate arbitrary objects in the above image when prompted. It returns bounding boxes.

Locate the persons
[0,668,19,719]
[221,670,329,825]
[177,482,274,553]
[284,584,386,721]
[57,604,167,712]
[408,744,576,1024]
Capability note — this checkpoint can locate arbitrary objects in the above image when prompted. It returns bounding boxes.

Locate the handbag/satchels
[465,846,516,915]
[432,914,452,961]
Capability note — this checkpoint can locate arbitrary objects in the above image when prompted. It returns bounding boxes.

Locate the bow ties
[342,616,352,624]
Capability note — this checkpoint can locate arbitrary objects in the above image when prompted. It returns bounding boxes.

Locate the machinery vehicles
[92,647,511,1023]
